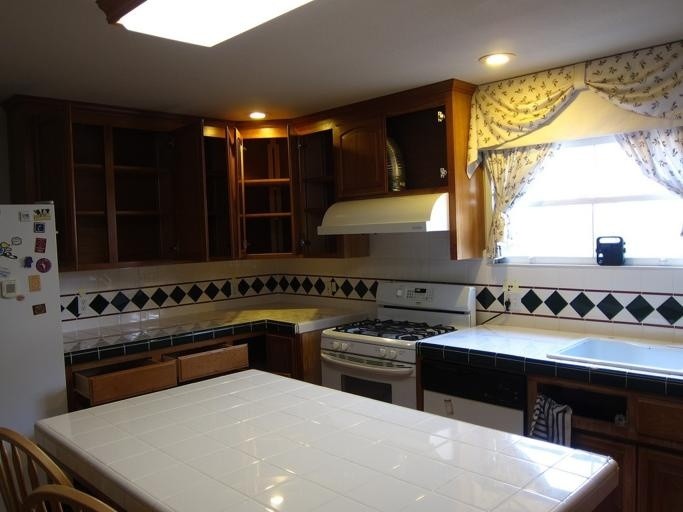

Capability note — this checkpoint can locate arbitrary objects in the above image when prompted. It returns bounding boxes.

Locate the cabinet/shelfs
[203,118,295,260]
[525,373,681,512]
[72,100,208,267]
[64,329,321,407]
[331,78,481,202]
[296,112,353,259]
[2,96,77,272]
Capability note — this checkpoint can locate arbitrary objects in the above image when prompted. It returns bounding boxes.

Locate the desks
[33,366,617,511]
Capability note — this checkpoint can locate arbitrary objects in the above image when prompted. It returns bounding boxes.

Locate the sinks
[546,337,683,377]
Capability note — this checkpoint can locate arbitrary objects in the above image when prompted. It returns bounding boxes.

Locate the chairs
[0,426,116,512]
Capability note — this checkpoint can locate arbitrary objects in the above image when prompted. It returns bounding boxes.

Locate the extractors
[317,193,450,235]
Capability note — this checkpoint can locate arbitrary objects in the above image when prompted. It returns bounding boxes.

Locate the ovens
[319,349,416,409]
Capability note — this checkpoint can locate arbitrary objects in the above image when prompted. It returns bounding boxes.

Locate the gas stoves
[320,317,458,363]
